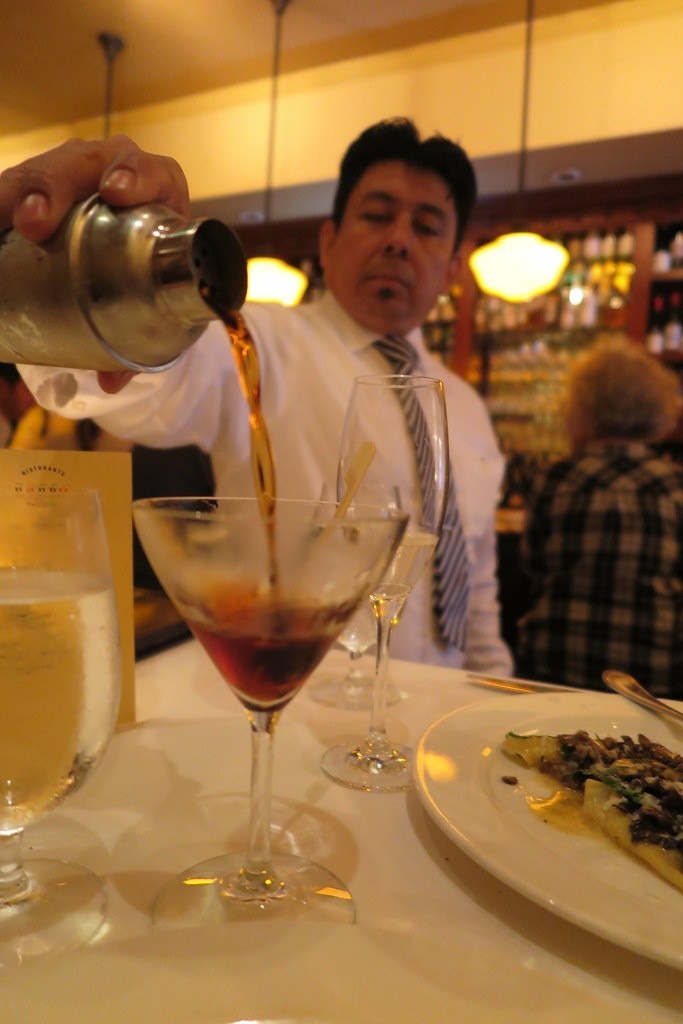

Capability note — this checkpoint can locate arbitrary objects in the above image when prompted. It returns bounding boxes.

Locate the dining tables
[1,638,682,1024]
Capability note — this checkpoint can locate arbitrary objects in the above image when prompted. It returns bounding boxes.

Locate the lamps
[245,0,309,308]
[469,0,569,305]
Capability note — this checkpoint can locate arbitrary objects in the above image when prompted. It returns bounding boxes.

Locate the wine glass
[130,497,409,942]
[0,488,123,959]
[323,375,448,792]
[311,481,403,710]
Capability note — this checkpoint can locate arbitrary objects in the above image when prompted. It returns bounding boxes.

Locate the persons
[0,118,512,680]
[503,333,683,701]
[7,404,134,452]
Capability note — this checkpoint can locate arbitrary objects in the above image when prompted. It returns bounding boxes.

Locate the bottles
[664,293,683,351]
[646,296,665,356]
[0,189,248,374]
[565,232,633,307]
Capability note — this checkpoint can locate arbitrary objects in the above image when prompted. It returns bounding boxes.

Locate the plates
[415,693,683,972]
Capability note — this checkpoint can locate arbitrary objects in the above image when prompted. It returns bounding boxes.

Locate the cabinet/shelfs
[231,171,683,463]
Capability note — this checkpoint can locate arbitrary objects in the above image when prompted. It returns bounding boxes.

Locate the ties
[374,329,473,652]
